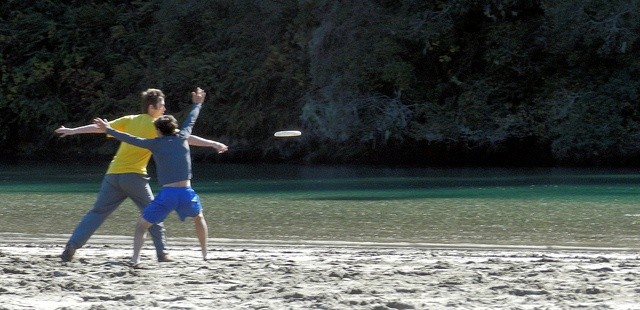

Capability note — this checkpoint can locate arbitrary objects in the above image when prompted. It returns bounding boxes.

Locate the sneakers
[158,249,175,262]
[63,246,76,261]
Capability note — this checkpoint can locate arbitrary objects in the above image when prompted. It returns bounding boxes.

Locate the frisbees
[274,131,301,137]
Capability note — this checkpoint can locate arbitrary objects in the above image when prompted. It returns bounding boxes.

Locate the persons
[92,87,208,263]
[55,88,229,262]
